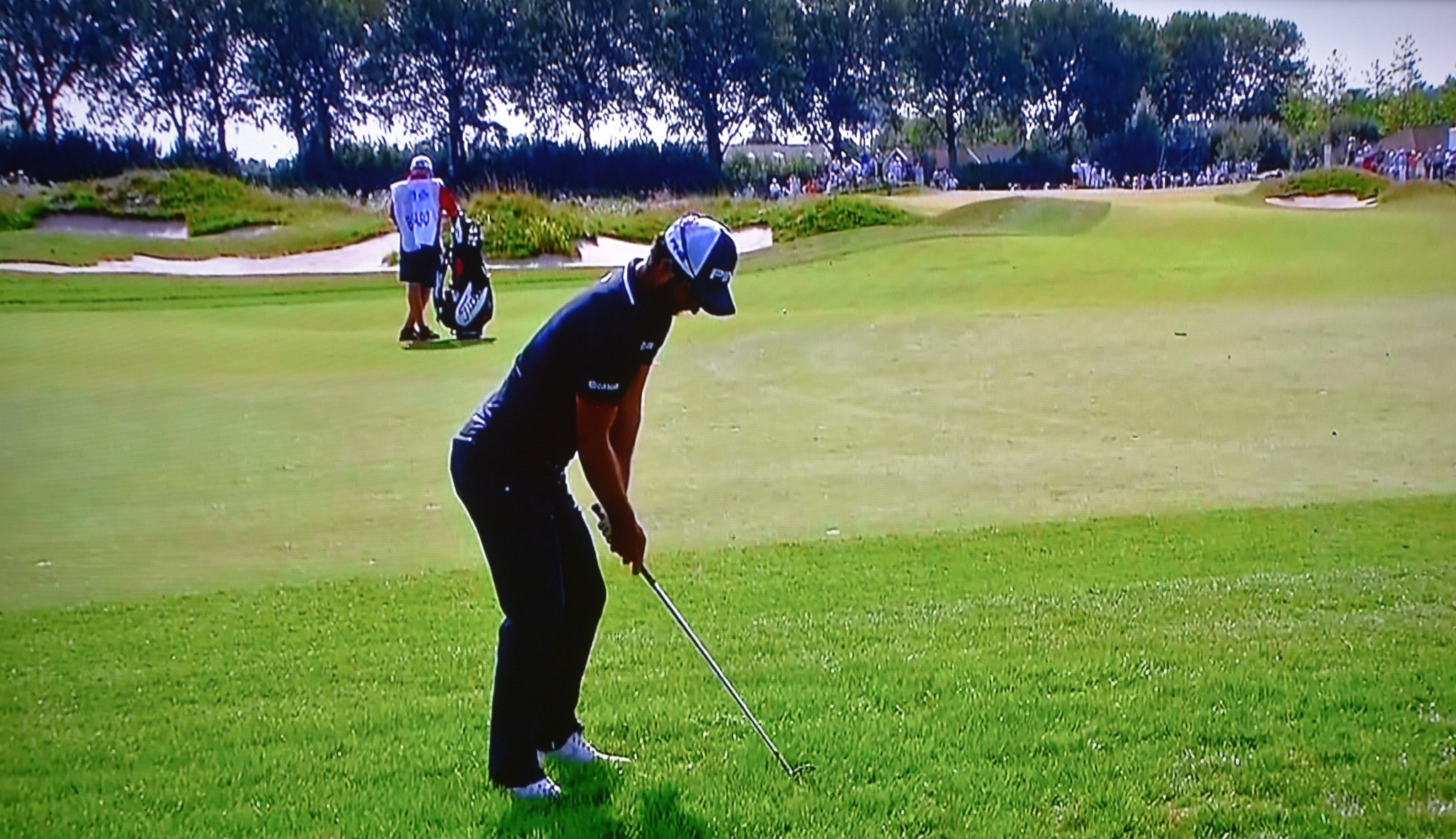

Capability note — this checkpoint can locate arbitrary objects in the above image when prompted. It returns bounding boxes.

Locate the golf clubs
[590,500,815,780]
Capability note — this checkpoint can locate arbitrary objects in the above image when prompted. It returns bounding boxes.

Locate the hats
[665,212,738,316]
[409,155,434,178]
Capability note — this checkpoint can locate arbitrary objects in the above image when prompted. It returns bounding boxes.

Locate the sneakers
[538,733,630,769]
[419,326,440,342]
[399,327,419,344]
[508,776,561,796]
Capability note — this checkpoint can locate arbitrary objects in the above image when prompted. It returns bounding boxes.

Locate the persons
[1067,154,1261,189]
[735,146,960,199]
[1346,133,1455,187]
[388,154,463,347]
[448,209,744,802]
